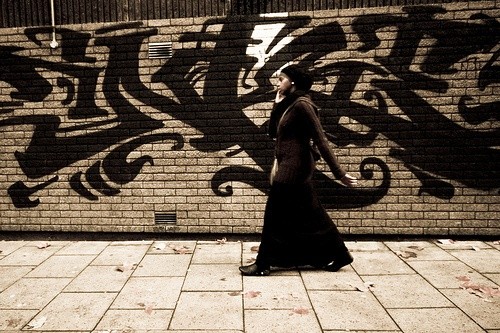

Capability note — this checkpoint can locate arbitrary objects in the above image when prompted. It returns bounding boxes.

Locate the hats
[281,65,313,93]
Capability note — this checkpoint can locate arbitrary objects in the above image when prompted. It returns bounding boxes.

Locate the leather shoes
[239,261,270,277]
[323,250,356,272]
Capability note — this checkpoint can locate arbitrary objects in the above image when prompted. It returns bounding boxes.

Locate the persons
[238,64,358,275]
